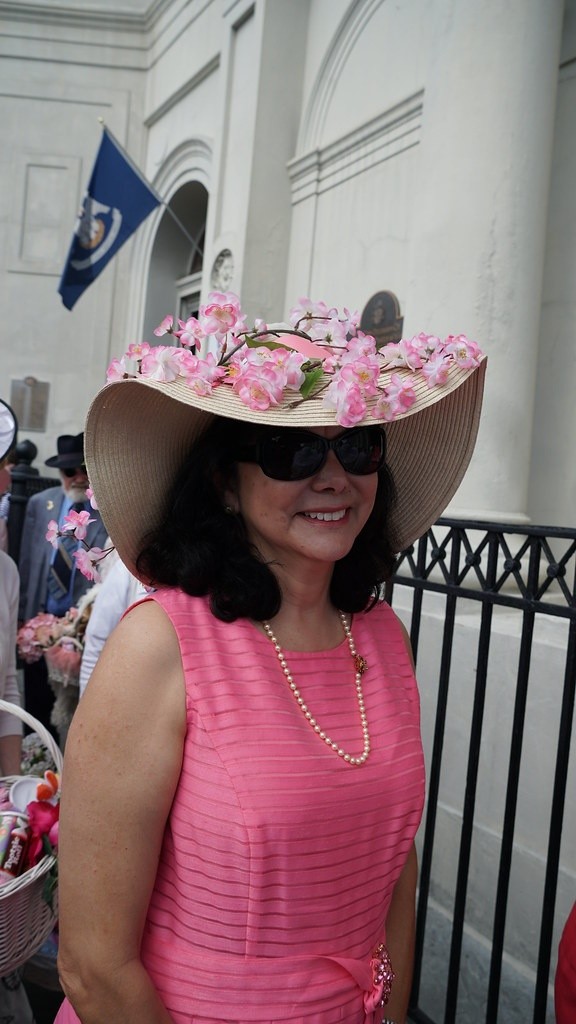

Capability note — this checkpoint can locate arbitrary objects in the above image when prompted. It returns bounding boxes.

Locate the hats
[45,432,86,469]
[83,337,489,592]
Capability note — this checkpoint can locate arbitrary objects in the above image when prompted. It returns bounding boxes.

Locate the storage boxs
[0,698,77,977]
[39,637,84,689]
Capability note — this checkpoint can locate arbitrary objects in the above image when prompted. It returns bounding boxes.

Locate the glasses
[61,466,87,476]
[231,426,386,482]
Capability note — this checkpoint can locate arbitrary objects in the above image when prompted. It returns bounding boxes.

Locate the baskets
[0,699,63,979]
[44,592,94,688]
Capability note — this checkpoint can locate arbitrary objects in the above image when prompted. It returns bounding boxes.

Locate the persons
[0,294,486,1024]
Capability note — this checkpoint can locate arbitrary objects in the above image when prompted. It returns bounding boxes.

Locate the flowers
[44,295,485,581]
[15,602,95,693]
[16,767,64,854]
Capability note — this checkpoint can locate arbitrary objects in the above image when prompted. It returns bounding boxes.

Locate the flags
[58,126,161,310]
